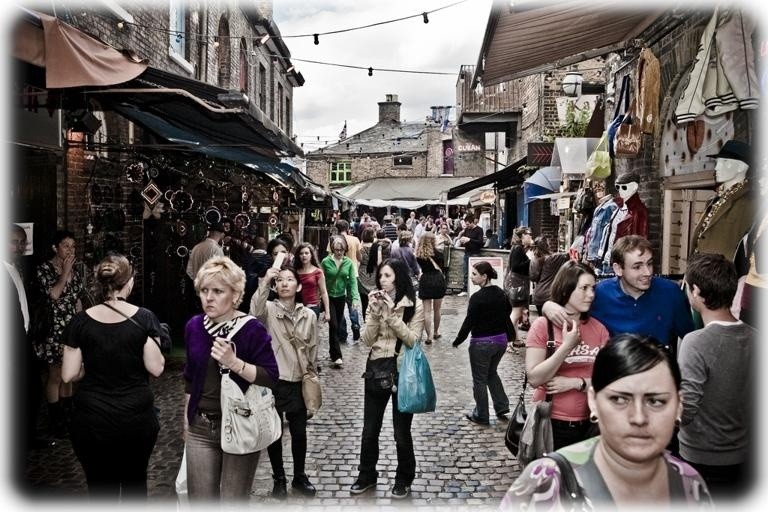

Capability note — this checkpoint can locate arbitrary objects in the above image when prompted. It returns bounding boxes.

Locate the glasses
[616,185,627,190]
[525,233,532,236]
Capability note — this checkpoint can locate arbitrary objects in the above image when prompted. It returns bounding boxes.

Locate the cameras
[374,291,386,300]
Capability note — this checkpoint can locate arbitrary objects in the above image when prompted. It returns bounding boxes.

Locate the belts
[306,304,317,308]
[551,418,589,428]
[198,410,221,424]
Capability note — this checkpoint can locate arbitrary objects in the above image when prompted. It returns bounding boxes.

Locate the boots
[48,396,74,439]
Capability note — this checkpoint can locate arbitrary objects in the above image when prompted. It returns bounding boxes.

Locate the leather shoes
[425,340,431,344]
[434,335,440,339]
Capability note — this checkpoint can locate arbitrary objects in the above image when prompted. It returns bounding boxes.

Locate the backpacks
[245,254,268,284]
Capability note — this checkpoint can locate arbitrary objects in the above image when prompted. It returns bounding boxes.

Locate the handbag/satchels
[607,115,631,159]
[174,443,188,511]
[504,292,516,342]
[320,298,325,312]
[410,277,420,291]
[32,261,54,343]
[159,323,173,354]
[570,235,585,261]
[301,373,322,413]
[585,130,612,181]
[505,397,537,461]
[361,355,397,391]
[397,335,436,414]
[572,178,596,214]
[508,285,529,307]
[613,97,642,158]
[221,374,282,454]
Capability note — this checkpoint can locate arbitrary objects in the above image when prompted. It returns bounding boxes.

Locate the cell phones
[272,252,285,279]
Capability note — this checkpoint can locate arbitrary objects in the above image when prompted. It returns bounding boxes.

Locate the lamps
[562,64,585,95]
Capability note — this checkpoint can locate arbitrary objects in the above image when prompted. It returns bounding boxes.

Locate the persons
[600,169,652,275]
[1,210,765,512]
[582,193,619,267]
[681,136,755,262]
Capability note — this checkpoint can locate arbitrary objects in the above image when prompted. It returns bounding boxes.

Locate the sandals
[513,340,525,347]
[506,345,519,354]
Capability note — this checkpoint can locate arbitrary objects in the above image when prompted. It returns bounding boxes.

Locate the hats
[706,140,754,167]
[616,173,640,184]
[384,215,392,221]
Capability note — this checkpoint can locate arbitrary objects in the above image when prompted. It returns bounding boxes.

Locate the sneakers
[292,473,315,496]
[350,479,376,494]
[518,322,529,331]
[457,291,467,297]
[392,480,411,499]
[466,414,489,425]
[353,328,360,340]
[273,475,287,500]
[497,410,509,417]
[334,358,342,367]
